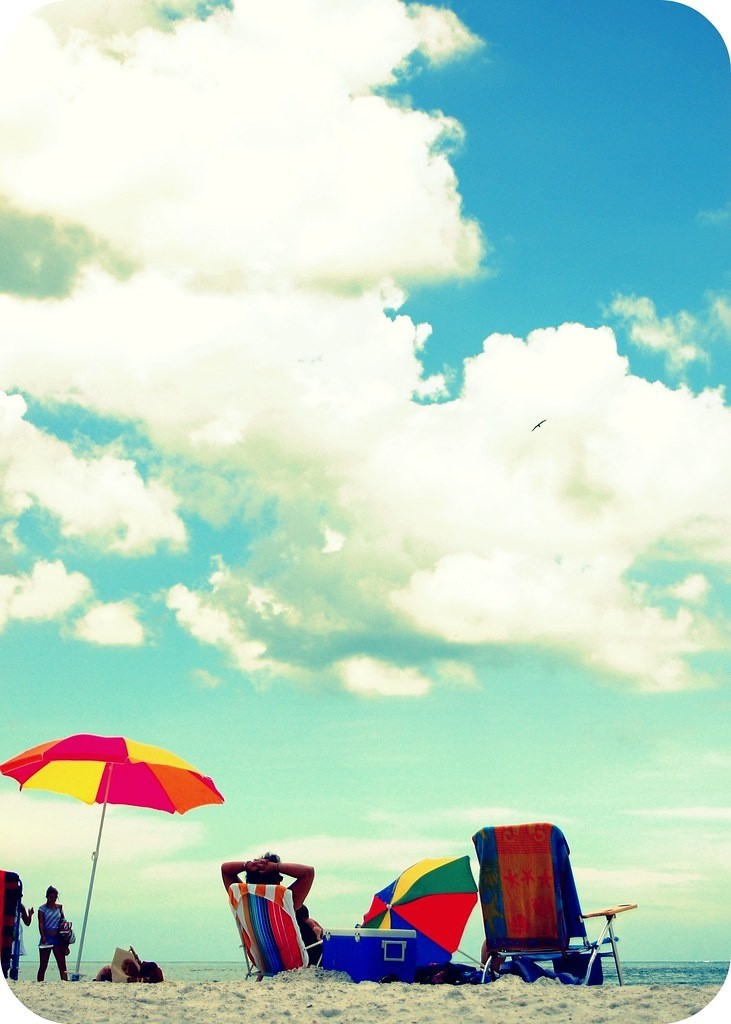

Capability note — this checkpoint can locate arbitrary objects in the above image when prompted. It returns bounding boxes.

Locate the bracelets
[244,861,250,869]
[276,863,278,871]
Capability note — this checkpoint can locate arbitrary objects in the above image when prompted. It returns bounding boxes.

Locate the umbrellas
[363,855,501,978]
[0,734,224,982]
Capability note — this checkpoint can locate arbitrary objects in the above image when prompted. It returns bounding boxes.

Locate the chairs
[228,882,323,982]
[472,822,639,986]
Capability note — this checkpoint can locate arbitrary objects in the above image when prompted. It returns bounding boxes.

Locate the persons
[221,853,323,967]
[10,894,35,969]
[481,940,520,975]
[37,886,70,981]
[93,954,163,983]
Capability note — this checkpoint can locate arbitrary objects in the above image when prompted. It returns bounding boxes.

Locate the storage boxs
[322,929,417,984]
